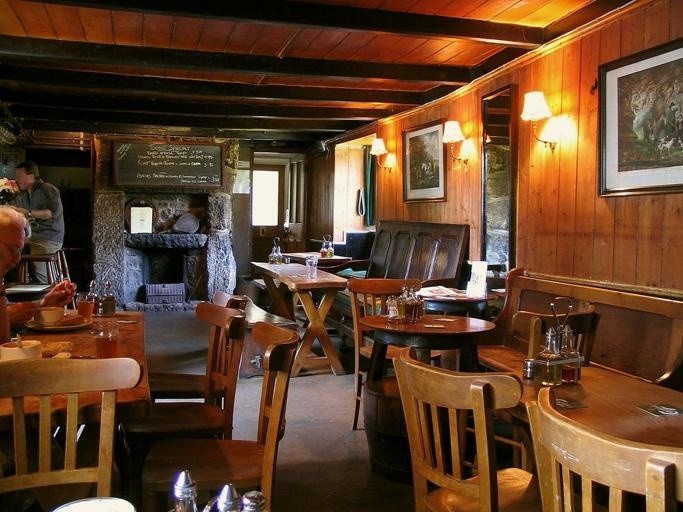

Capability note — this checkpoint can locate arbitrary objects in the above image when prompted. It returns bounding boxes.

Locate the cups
[94,323,119,359]
[77,300,93,317]
[102,300,115,316]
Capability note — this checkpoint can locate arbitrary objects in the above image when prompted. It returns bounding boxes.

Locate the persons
[0,205,78,345]
[5,159,65,286]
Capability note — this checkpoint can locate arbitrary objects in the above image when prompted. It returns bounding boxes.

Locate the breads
[57,315,83,326]
[41,341,73,358]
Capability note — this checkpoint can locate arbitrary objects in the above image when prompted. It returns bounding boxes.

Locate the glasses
[0,240,23,256]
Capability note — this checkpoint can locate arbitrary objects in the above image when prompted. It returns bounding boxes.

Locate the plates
[26,317,94,331]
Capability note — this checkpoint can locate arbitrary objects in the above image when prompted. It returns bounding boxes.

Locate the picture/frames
[402,118,446,203]
[599,38,683,197]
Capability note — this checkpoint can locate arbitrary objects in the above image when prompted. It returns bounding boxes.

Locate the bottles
[319,243,327,257]
[397,285,406,318]
[327,242,334,258]
[536,327,562,386]
[523,358,533,380]
[275,246,282,264]
[405,288,419,320]
[304,256,318,280]
[269,247,276,263]
[387,295,397,321]
[284,258,291,263]
[168,468,268,512]
[562,325,581,383]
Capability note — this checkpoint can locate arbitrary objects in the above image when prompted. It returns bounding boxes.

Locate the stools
[19,249,76,311]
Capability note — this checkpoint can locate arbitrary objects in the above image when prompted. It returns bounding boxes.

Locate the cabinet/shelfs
[111,138,226,190]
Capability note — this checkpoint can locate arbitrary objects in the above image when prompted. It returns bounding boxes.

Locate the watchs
[28,208,32,218]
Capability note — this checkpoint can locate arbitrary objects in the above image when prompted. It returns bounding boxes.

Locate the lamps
[371,138,392,172]
[441,120,468,166]
[521,91,558,153]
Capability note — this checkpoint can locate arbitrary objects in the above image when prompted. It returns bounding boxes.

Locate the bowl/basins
[35,307,63,321]
[0,340,42,361]
